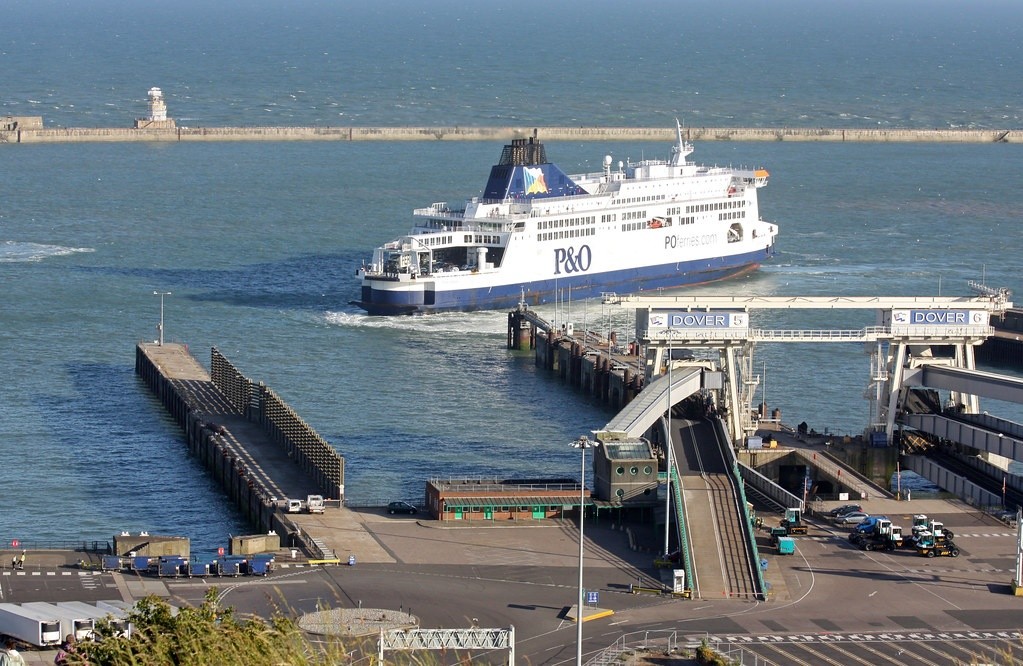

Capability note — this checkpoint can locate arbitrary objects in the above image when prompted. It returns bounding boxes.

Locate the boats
[348,116,781,318]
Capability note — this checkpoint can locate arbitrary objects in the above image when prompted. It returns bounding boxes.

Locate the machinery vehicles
[848,515,960,559]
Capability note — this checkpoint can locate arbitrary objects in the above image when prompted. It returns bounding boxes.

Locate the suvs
[831,504,862,517]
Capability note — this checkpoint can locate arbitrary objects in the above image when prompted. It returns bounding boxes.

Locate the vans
[285,499,302,512]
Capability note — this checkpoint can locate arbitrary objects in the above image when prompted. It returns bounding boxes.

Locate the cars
[836,511,870,523]
[386,501,417,515]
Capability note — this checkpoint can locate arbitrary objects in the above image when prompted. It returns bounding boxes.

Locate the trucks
[769,526,795,555]
[780,508,808,535]
[306,495,325,514]
